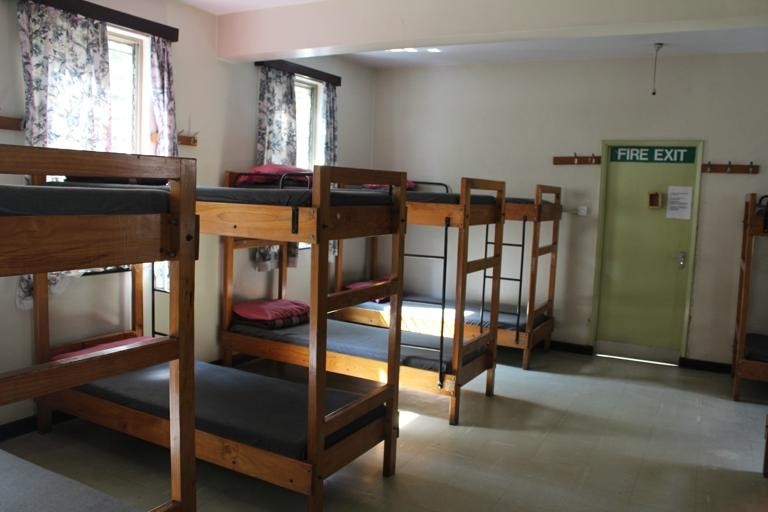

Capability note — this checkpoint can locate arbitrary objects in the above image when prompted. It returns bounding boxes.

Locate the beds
[731,192,768,402]
[33,165,407,512]
[3,143,199,511]
[218,163,561,425]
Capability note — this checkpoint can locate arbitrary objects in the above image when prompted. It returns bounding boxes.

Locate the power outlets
[576,205,588,217]
